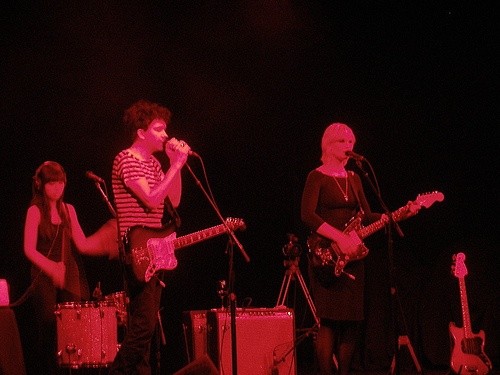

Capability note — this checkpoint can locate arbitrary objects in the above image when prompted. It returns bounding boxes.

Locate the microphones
[346,150,364,161]
[85,171,104,183]
[164,140,197,156]
[288,232,299,243]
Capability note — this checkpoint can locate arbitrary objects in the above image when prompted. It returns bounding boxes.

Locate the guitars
[448,252,492,375]
[306,191,445,267]
[122,217,246,284]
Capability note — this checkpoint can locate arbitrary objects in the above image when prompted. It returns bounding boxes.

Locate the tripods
[275,251,340,371]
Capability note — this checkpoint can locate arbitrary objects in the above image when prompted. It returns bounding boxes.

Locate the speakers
[179,305,298,375]
[56,299,120,369]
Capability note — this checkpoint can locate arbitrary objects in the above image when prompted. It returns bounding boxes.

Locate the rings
[181,142,184,146]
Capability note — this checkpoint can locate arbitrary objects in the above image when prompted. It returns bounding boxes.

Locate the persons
[112,104,189,375]
[302,123,420,375]
[24,161,103,375]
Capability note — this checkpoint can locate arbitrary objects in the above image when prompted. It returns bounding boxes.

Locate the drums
[102,290,166,349]
[53,299,120,369]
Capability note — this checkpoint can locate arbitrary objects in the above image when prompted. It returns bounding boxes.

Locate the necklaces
[325,165,349,200]
